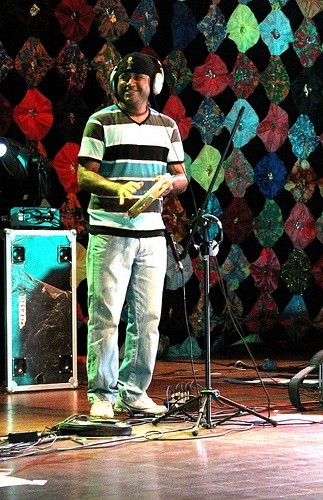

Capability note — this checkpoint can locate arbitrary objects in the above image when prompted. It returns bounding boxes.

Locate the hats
[117,54,154,78]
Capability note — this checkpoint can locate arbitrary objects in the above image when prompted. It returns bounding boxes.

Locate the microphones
[161,215,183,272]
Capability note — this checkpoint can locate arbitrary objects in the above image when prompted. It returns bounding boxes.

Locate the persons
[0,93,45,229]
[76,50,189,419]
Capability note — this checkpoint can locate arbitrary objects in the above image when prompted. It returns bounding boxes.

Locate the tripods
[152,106,279,436]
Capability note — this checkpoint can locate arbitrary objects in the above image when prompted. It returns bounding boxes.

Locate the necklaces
[119,102,148,116]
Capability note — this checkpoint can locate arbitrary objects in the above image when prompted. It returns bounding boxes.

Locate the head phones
[189,214,224,260]
[109,55,164,96]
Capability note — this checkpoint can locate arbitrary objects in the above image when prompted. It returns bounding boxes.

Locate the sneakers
[90,401,114,419]
[114,392,167,414]
